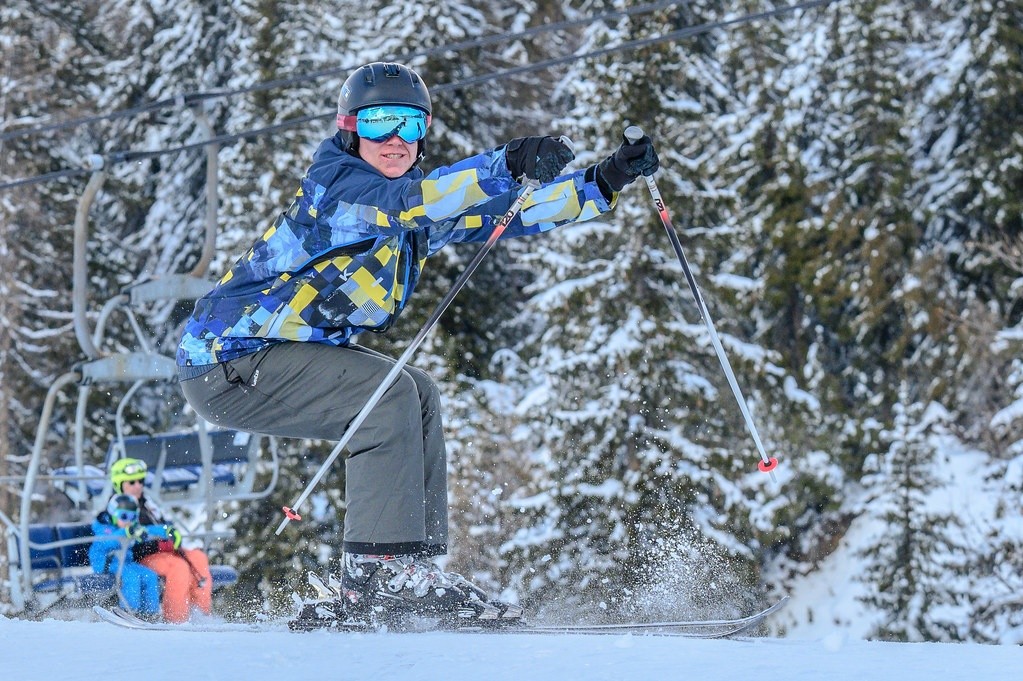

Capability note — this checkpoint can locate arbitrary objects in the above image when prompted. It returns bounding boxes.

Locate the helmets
[337,62,432,144]
[107,493,141,525]
[111,458,148,495]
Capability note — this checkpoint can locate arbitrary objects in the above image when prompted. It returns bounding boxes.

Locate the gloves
[166,525,183,550]
[505,135,576,183]
[125,525,148,542]
[599,125,659,191]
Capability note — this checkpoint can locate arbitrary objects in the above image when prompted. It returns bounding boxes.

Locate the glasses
[123,459,148,474]
[129,479,144,485]
[118,511,138,522]
[357,105,429,144]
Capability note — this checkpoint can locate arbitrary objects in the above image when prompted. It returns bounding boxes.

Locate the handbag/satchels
[132,540,159,563]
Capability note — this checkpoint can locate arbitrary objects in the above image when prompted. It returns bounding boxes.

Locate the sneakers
[337,552,523,633]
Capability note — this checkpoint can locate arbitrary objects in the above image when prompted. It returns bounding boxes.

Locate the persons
[87,457,212,622]
[178,60,660,624]
